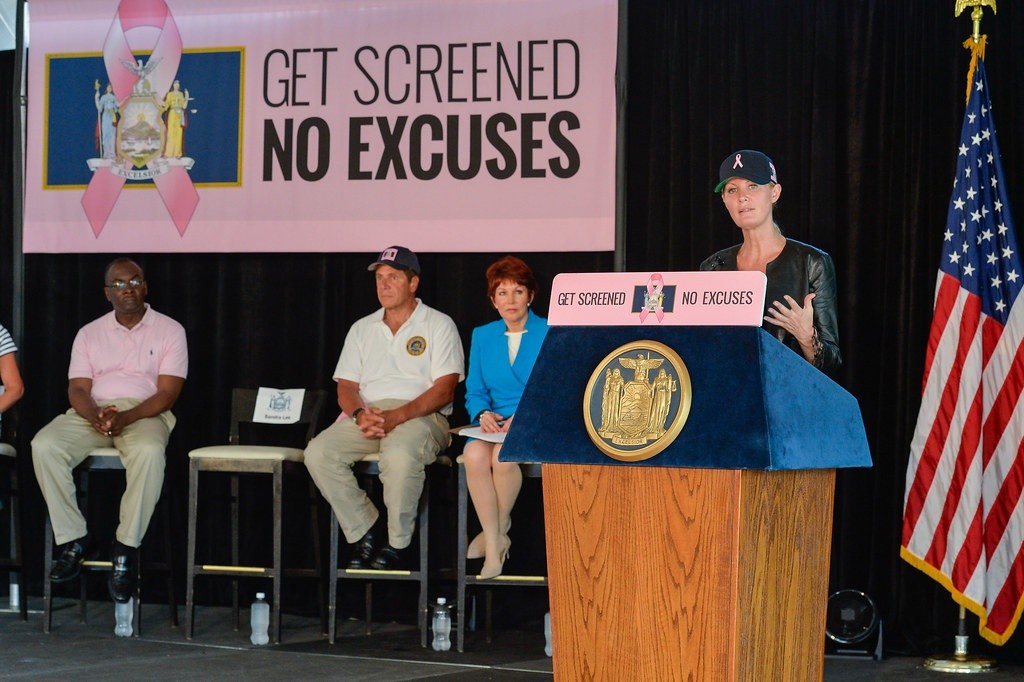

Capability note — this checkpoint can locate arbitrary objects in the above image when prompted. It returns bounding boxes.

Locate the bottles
[113,596,134,637]
[250,592,270,645]
[543,611,554,657]
[430,597,451,651]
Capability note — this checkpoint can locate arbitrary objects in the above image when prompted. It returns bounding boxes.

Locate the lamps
[824,588,881,661]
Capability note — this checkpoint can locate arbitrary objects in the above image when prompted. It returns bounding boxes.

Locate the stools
[327,453,454,648]
[0,388,27,618]
[43,446,176,638]
[185,389,328,647]
[455,457,547,652]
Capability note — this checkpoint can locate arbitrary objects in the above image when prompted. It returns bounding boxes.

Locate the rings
[109,431,112,436]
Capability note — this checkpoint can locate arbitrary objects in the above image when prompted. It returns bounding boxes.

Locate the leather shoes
[369,532,420,571]
[109,537,139,603]
[48,532,100,582]
[345,512,383,569]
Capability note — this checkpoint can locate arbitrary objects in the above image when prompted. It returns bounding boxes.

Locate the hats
[368,246,425,281]
[714,150,777,193]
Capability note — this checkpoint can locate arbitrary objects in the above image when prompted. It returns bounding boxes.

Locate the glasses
[104,279,145,290]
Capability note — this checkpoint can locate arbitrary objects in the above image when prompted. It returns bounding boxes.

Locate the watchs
[352,408,364,425]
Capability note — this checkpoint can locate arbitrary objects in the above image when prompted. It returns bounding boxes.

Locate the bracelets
[479,412,494,425]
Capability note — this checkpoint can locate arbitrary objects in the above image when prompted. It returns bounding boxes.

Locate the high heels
[476,534,511,580]
[467,515,511,559]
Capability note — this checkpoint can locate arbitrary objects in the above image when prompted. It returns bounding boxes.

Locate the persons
[305,244,465,569]
[0,325,23,440]
[30,261,188,603]
[463,258,549,579]
[700,150,842,376]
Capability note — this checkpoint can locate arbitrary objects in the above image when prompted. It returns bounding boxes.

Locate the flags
[900,36,1024,644]
